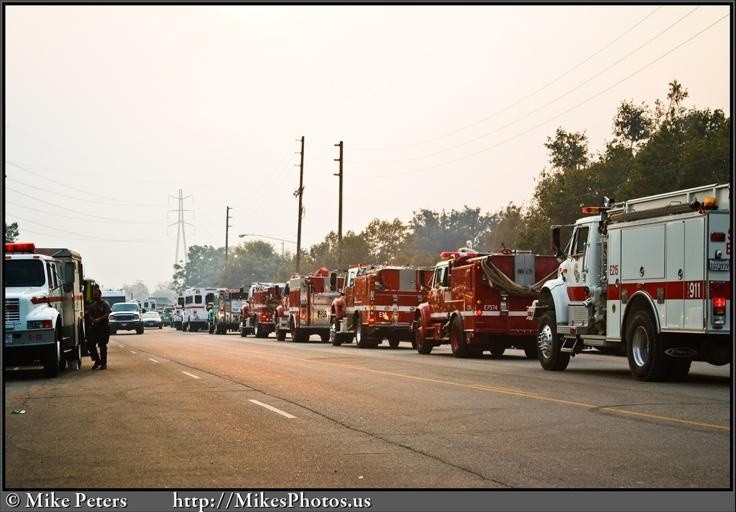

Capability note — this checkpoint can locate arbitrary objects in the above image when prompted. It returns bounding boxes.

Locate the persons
[83,286,110,371]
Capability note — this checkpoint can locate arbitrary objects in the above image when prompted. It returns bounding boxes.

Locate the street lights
[238,234,284,259]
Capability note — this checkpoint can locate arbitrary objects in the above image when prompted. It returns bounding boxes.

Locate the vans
[164,307,175,328]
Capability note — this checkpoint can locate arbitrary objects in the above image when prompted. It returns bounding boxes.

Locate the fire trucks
[5,243,88,378]
[535,182,730,382]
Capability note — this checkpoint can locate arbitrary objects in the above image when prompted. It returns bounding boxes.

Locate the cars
[100,288,164,335]
